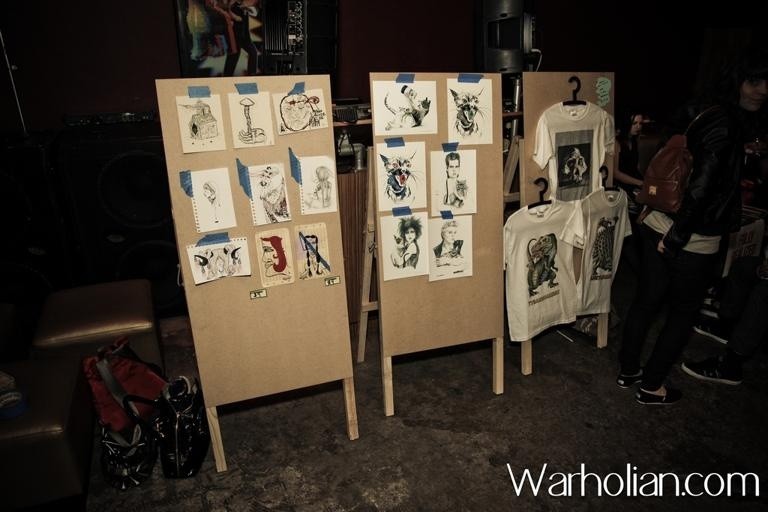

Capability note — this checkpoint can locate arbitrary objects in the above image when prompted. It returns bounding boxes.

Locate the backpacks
[638,103,720,215]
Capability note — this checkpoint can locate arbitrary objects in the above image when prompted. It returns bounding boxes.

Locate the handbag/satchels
[161,376,211,478]
[101,417,158,491]
[82,336,168,433]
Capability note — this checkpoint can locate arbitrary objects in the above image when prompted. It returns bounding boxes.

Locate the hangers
[526,174,551,211]
[562,74,586,106]
[597,162,618,195]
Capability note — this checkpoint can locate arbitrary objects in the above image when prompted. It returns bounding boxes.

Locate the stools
[31,276,165,384]
[1,358,92,512]
[137,343,139,345]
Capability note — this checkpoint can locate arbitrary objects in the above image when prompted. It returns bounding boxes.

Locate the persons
[201,1,259,76]
[442,152,467,208]
[390,218,425,272]
[680,249,768,387]
[615,107,647,214]
[612,46,768,406]
[433,221,463,267]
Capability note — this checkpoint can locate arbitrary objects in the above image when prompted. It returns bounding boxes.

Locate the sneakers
[635,386,683,406]
[693,319,729,345]
[680,356,743,386]
[615,366,647,388]
